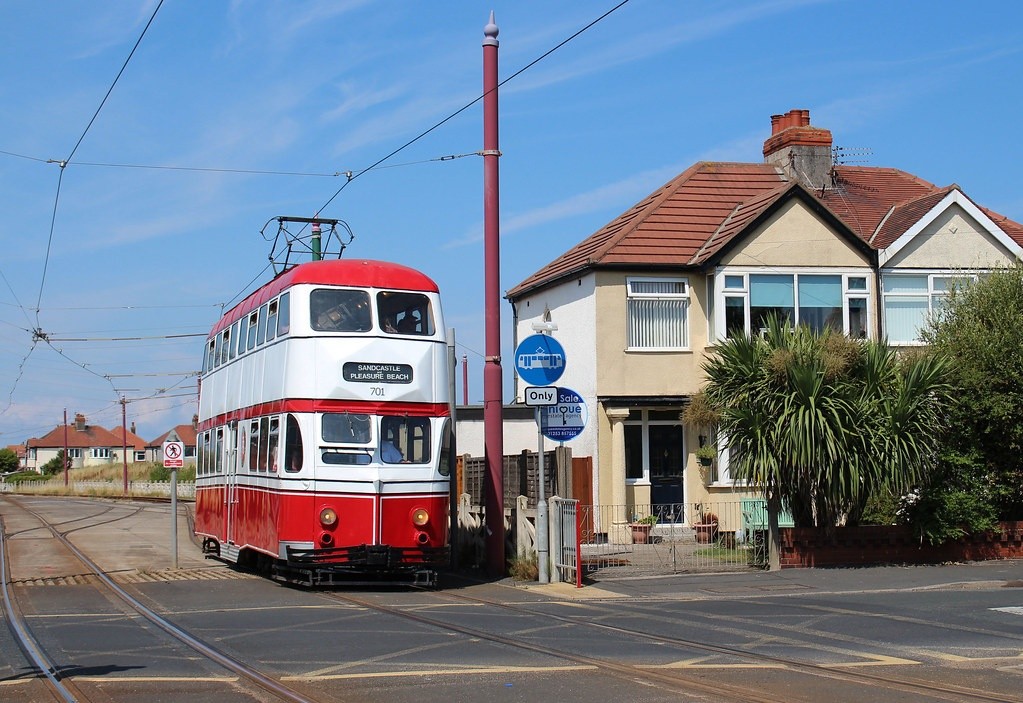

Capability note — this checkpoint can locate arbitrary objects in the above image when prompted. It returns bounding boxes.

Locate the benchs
[739,497,795,547]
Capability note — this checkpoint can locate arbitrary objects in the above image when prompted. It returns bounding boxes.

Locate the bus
[189,214,472,595]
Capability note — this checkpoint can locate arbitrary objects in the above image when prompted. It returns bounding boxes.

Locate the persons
[356,426,413,464]
[262,438,300,471]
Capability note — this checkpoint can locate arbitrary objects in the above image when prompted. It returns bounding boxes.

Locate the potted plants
[629,511,659,544]
[692,502,719,545]
[696,445,717,466]
